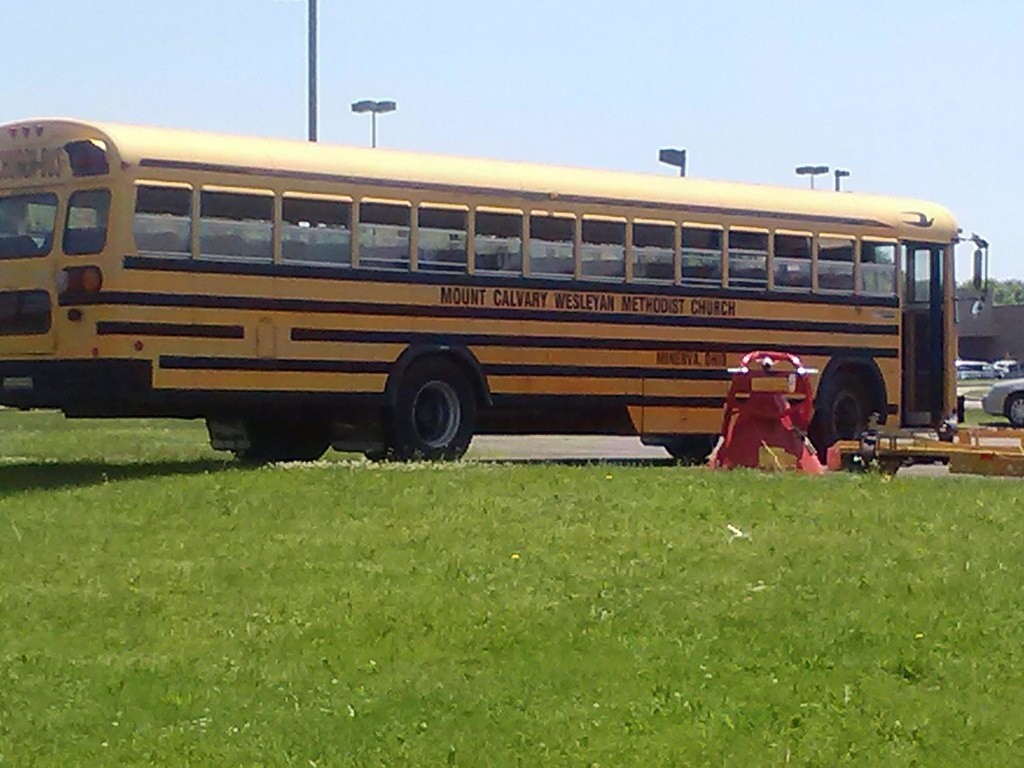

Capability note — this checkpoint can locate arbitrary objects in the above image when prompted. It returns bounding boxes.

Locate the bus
[0,117,989,464]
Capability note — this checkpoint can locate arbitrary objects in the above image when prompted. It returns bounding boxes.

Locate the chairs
[0,228,869,295]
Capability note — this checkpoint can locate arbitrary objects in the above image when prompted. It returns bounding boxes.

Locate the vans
[955,360,1024,379]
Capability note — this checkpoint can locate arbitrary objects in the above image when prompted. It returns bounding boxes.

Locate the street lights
[351,100,398,148]
[791,164,832,188]
[657,148,688,178]
[832,168,851,191]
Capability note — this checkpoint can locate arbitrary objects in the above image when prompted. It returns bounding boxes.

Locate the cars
[979,377,1024,427]
